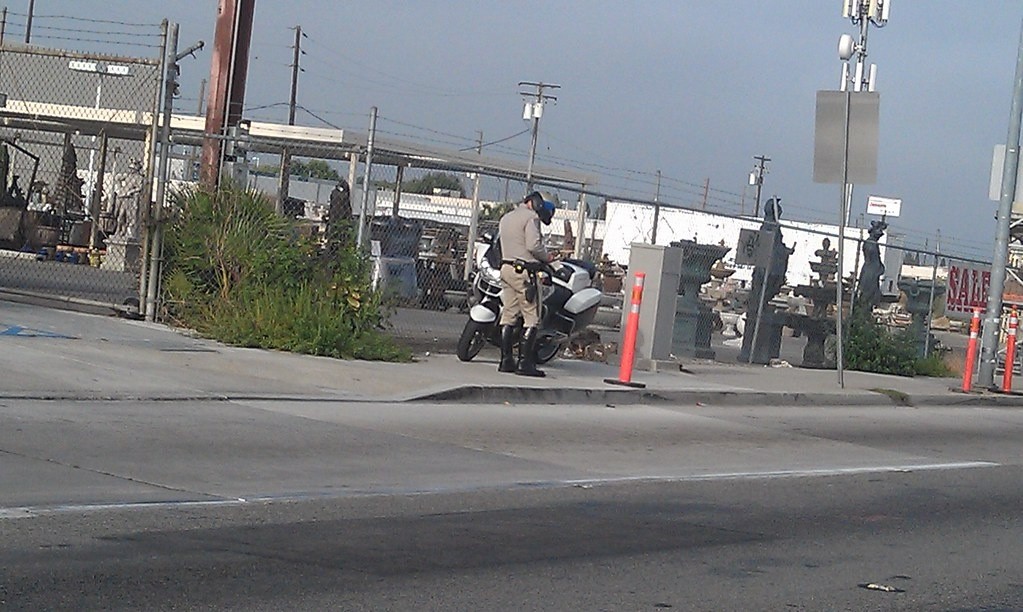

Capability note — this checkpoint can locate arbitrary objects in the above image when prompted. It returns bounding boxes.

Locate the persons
[858,220,886,306]
[498,191,554,377]
[113,161,143,238]
[748,197,795,311]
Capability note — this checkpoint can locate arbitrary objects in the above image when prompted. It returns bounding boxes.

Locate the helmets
[524,191,555,225]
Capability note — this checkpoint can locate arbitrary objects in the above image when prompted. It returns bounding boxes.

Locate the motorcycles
[456,230,604,365]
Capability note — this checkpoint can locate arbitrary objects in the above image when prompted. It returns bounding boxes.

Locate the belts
[503,261,541,271]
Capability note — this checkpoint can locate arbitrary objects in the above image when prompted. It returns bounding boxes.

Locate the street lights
[69,60,130,222]
[836,0,891,229]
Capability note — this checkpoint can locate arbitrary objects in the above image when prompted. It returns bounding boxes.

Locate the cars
[369,215,471,289]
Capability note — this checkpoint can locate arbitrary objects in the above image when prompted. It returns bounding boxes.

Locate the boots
[498,325,517,372]
[515,327,544,376]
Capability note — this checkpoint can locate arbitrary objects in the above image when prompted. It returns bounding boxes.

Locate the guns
[525,280,537,303]
